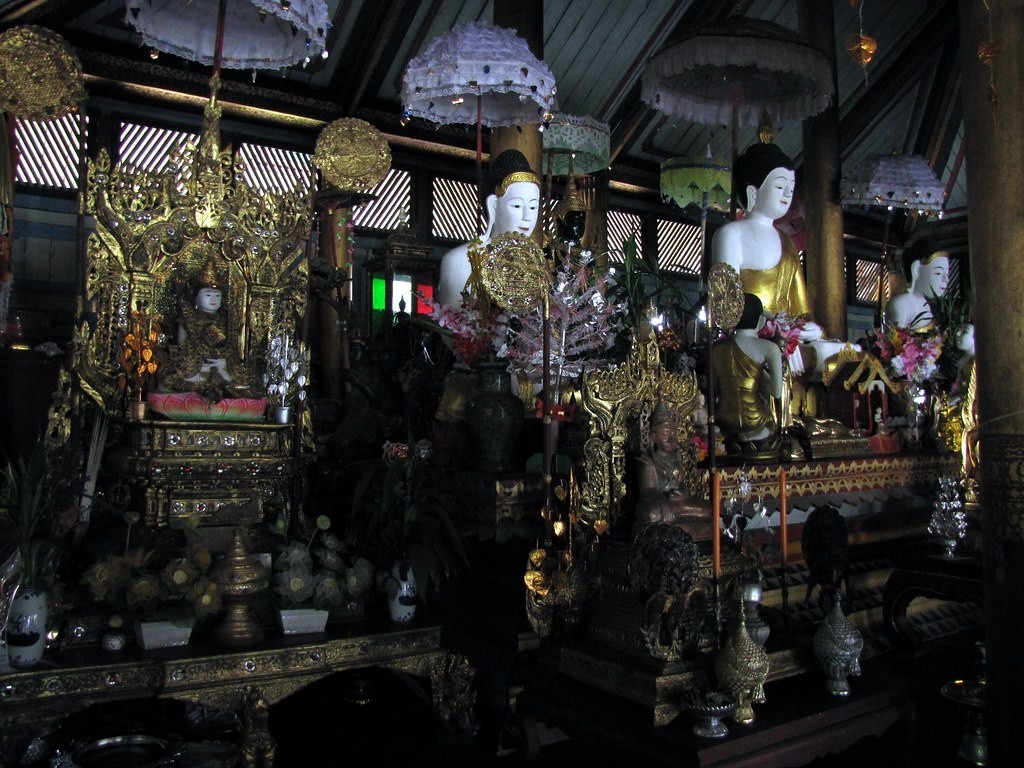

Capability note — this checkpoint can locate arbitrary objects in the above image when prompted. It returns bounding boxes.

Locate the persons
[170,256,234,383]
[634,388,713,524]
[883,232,978,420]
[394,296,410,323]
[434,149,583,424]
[705,144,862,434]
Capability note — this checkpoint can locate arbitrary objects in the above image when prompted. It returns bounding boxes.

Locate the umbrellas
[660,144,737,215]
[399,19,557,172]
[541,112,611,178]
[732,196,807,254]
[639,12,836,224]
[126,0,335,80]
[838,146,947,263]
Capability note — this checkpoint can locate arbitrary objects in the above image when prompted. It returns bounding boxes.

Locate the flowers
[415,270,506,361]
[866,319,943,386]
[759,313,805,358]
